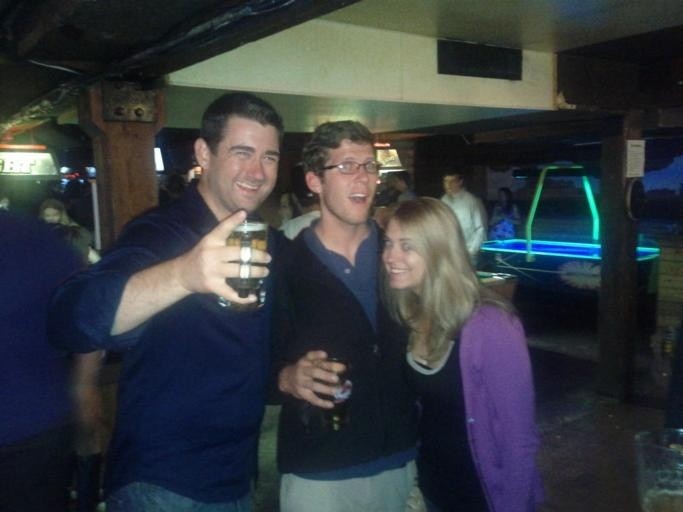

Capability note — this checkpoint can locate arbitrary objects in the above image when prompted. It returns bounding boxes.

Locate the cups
[314,356,351,433]
[633,427,682,511]
[216,219,269,312]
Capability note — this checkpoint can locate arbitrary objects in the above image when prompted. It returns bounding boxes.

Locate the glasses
[324,160,379,175]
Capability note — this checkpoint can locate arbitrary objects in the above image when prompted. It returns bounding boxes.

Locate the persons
[381,196,545,512]
[1,195,102,512]
[38,178,98,264]
[263,120,426,512]
[46,91,294,512]
[279,164,520,274]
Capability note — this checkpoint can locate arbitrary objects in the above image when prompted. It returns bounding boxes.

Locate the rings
[239,264,250,278]
[241,247,250,262]
